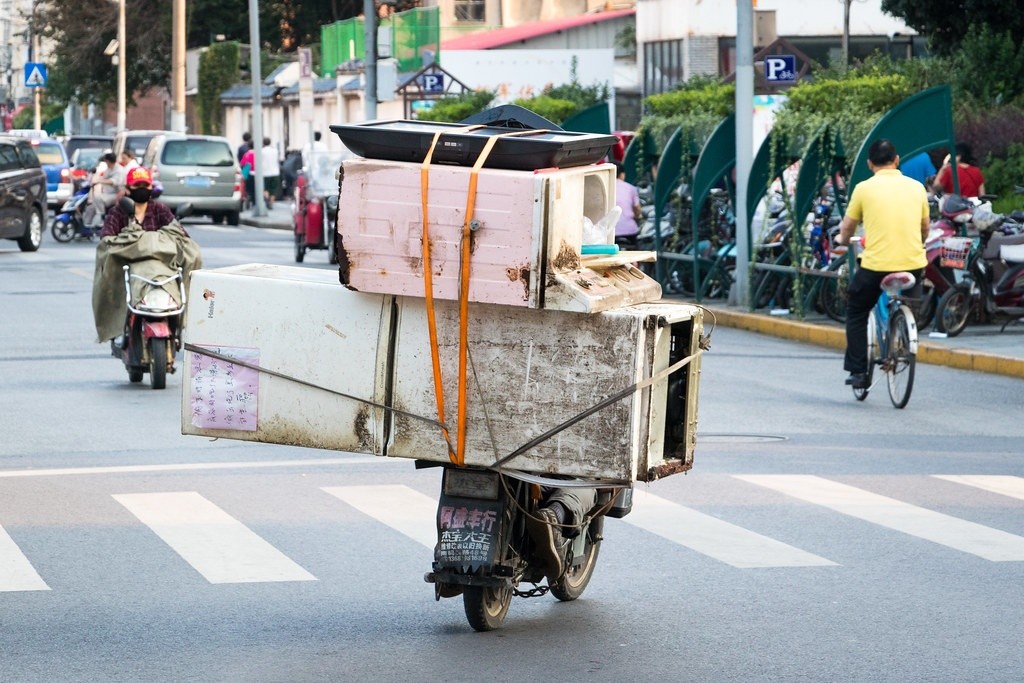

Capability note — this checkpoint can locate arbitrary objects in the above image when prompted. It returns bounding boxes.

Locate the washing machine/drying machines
[334,159,663,315]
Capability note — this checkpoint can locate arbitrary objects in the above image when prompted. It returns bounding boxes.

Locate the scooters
[110,197,186,390]
[634,174,1024,337]
[51,168,108,243]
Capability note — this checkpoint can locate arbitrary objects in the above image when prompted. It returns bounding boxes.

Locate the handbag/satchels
[242,152,250,179]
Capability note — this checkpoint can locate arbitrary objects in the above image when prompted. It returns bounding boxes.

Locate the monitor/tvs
[328,119,621,171]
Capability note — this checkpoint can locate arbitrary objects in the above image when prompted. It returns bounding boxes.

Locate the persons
[615,162,644,267]
[237,131,329,211]
[82,150,141,229]
[100,167,190,239]
[932,144,988,284]
[835,139,931,389]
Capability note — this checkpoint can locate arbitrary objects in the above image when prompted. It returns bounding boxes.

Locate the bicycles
[834,236,918,409]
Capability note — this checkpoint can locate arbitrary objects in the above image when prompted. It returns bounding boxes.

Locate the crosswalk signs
[25,62,48,87]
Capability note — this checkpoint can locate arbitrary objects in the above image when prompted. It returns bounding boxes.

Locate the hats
[127,167,153,185]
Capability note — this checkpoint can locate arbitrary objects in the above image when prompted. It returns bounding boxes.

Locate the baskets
[940,236,971,269]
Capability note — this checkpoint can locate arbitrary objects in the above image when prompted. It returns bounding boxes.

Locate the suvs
[114,131,170,165]
[141,134,243,225]
[0,132,47,251]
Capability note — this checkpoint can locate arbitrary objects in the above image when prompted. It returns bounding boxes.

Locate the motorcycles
[294,147,341,263]
[414,454,605,632]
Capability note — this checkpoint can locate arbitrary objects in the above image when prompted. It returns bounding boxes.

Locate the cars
[29,139,76,214]
[59,136,116,168]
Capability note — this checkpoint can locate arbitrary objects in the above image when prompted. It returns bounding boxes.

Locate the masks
[125,186,153,204]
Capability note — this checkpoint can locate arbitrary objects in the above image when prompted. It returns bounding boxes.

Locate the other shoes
[114,334,124,348]
[95,221,104,228]
[75,234,88,240]
[852,376,869,386]
[264,197,273,209]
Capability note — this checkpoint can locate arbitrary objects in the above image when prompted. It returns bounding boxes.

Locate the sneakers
[526,509,566,579]
